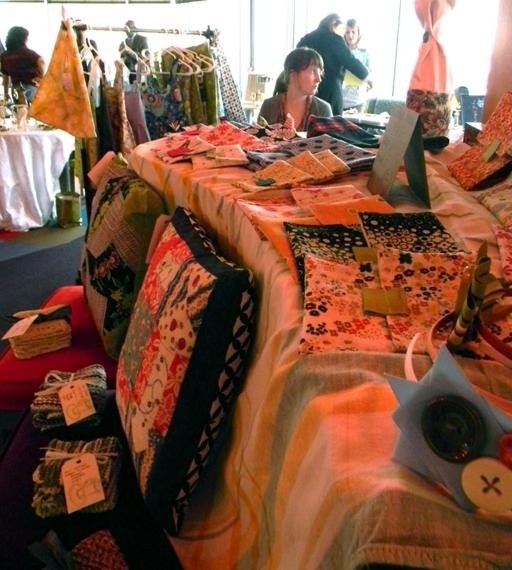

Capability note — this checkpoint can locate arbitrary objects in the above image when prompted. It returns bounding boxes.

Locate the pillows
[80,153,170,360]
[116,204,260,538]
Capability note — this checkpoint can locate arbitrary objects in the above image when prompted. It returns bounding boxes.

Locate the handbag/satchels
[55,191,81,229]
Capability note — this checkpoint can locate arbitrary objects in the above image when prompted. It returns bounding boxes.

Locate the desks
[342,98,363,113]
[0,112,76,232]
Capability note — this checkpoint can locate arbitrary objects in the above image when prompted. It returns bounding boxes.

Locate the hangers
[68,19,217,79]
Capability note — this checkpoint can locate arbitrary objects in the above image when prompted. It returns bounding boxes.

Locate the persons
[295,13,372,116]
[343,17,371,111]
[1,26,45,105]
[76,20,105,80]
[255,46,332,132]
[119,20,149,84]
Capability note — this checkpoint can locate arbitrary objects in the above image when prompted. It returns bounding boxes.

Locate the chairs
[373,97,405,115]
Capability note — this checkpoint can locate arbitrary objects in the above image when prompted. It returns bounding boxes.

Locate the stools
[0,286,118,412]
